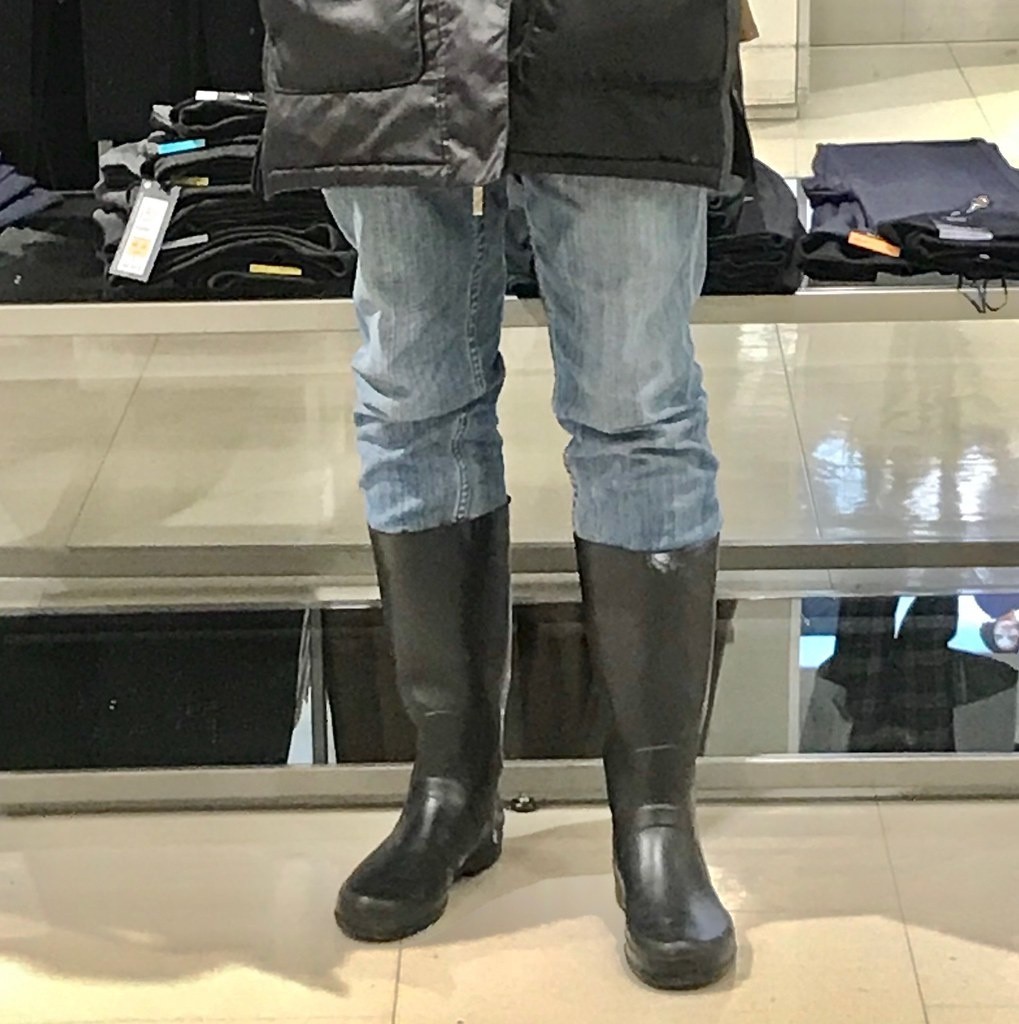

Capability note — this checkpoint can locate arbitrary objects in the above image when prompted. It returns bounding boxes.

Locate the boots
[576,539,736,990]
[335,501,511,941]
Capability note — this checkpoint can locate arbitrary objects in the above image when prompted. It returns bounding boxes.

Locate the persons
[972,592,1019,654]
[254,0,755,994]
[797,595,1018,758]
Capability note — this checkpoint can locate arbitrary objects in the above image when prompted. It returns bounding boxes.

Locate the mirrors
[0,587,1019,813]
